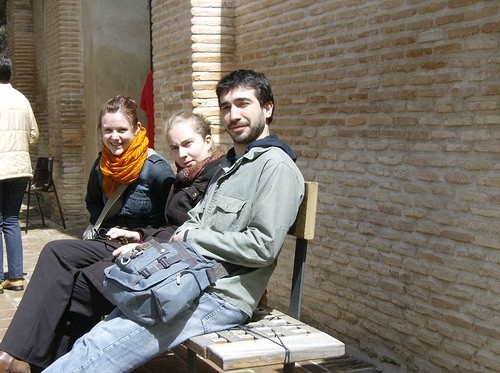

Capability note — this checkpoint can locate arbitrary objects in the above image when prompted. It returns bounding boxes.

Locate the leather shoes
[0,350,31,373]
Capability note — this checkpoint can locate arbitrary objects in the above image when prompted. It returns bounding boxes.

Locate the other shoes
[0,276,24,294]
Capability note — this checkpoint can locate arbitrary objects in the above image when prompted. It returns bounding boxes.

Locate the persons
[0,54,39,294]
[0,68,307,373]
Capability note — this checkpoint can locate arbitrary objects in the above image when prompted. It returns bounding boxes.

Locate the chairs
[24,157,67,234]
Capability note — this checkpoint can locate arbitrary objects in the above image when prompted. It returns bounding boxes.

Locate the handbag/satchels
[82,222,97,239]
[102,239,218,326]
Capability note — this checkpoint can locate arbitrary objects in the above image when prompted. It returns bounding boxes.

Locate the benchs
[172,181,346,373]
